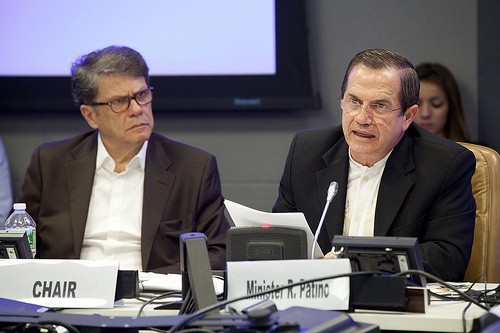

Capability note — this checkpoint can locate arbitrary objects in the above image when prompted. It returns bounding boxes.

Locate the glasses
[338,96,403,114]
[86,87,154,114]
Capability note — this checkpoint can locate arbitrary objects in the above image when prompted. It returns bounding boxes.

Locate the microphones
[311,181,338,258]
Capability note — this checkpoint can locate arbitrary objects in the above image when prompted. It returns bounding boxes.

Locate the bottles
[4,202,36,259]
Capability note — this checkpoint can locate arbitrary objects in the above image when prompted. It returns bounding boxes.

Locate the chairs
[427,141,500,283]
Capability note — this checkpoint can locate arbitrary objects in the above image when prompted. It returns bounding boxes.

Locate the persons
[412,62,470,143]
[2,46,232,291]
[272,49,477,287]
